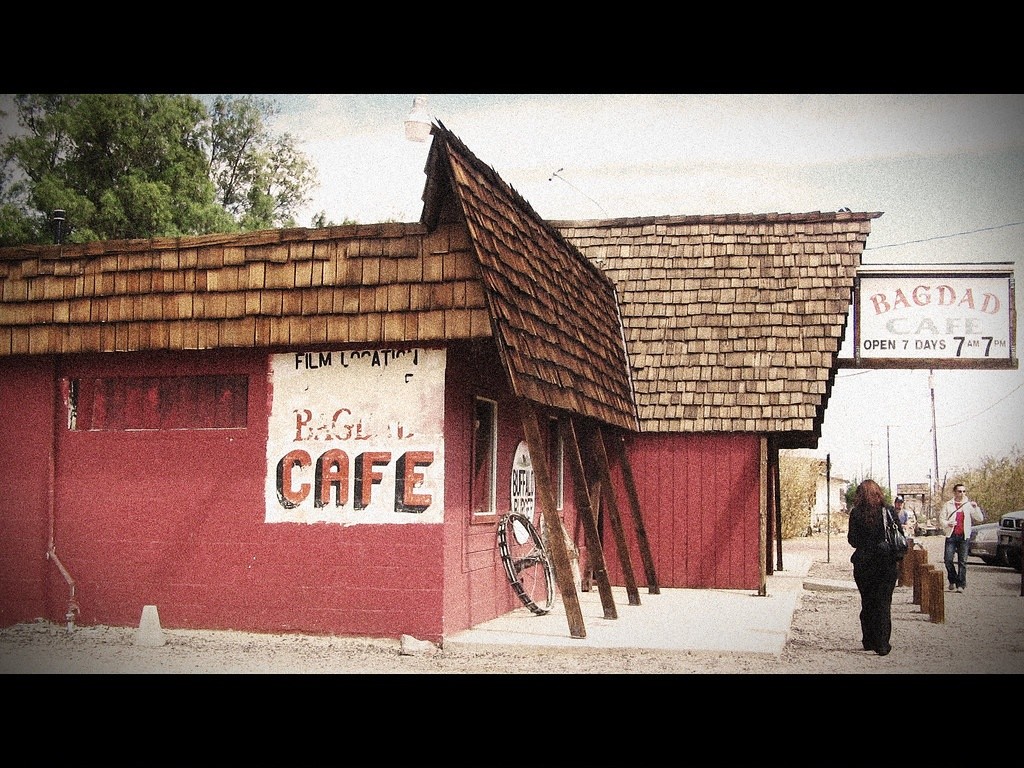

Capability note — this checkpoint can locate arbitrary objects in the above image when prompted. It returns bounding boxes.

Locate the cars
[967,521,1001,565]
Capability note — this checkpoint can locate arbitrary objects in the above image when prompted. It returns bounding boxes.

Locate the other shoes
[861,639,891,656]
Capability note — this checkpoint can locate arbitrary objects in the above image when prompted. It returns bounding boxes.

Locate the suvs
[998,510,1024,569]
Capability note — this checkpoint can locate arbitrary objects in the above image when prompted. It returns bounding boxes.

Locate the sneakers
[948,583,965,593]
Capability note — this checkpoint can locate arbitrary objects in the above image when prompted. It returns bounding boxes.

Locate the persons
[848,478,916,657]
[893,494,909,535]
[939,483,984,593]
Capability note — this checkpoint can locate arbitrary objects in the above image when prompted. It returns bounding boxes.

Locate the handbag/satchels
[877,508,908,568]
